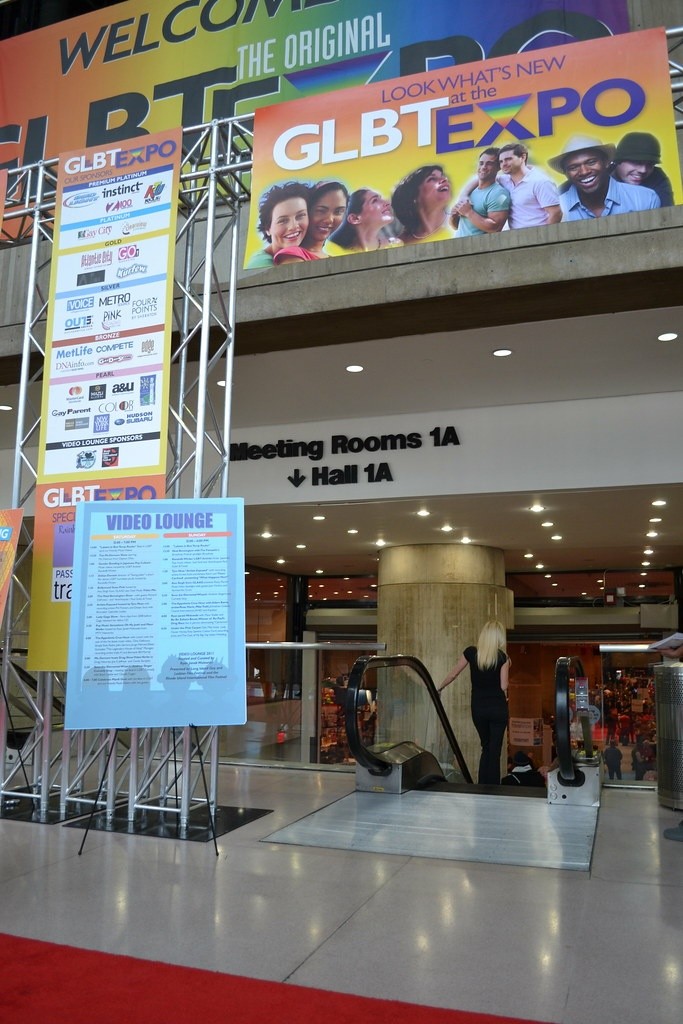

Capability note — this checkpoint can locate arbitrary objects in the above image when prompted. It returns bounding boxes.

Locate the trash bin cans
[651,660,683,811]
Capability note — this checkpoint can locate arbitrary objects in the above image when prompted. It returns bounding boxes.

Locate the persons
[246,131,675,271]
[435,622,510,785]
[275,728,286,758]
[591,674,656,780]
[648,642,683,663]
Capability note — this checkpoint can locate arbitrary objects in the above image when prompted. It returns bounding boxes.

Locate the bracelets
[436,687,441,692]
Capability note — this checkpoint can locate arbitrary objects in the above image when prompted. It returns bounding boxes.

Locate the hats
[512,751,530,766]
